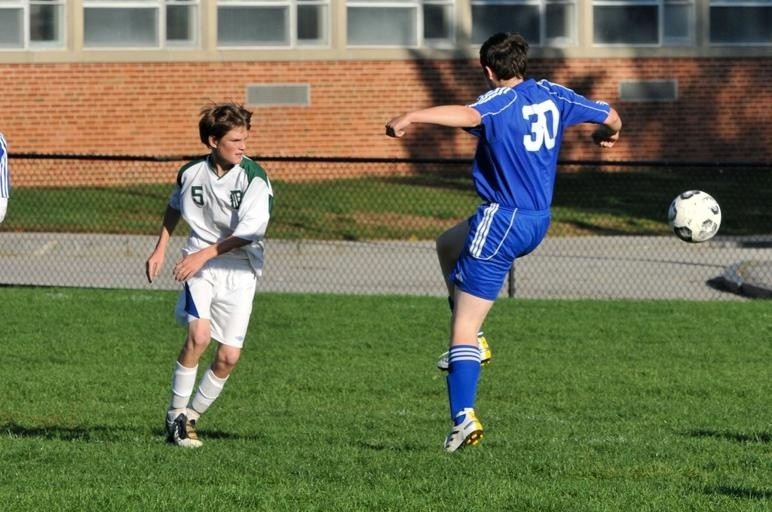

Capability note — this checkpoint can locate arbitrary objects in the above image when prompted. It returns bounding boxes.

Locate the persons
[144,101,273,449]
[385,31,623,457]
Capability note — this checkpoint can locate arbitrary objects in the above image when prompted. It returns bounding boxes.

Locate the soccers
[669,190,721,244]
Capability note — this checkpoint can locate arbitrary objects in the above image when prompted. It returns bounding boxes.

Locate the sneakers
[165,409,207,450]
[435,330,494,375]
[442,404,485,456]
